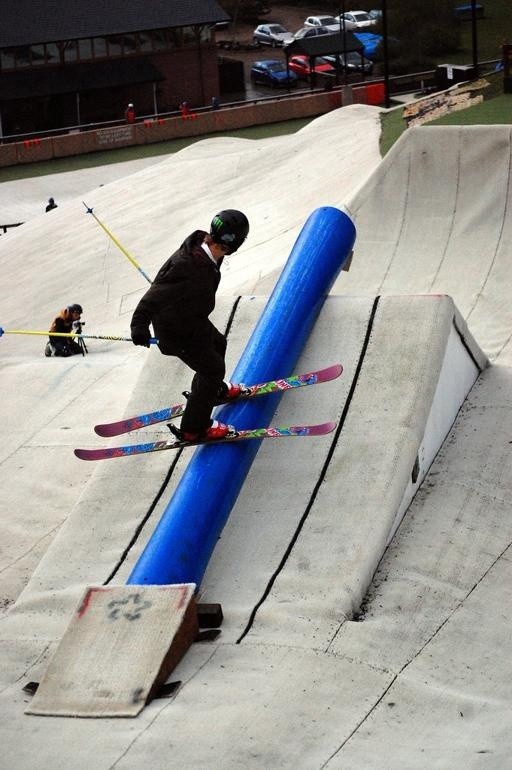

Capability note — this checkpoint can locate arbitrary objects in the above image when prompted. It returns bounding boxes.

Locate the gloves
[131,328,151,348]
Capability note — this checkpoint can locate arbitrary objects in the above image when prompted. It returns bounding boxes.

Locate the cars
[250,10,383,90]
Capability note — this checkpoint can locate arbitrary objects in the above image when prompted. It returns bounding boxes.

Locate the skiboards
[74,365,343,460]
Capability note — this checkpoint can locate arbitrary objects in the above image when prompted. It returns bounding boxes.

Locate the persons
[45,198,58,213]
[130,209,252,443]
[43,303,84,357]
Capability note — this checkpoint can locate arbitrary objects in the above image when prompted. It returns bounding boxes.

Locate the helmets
[70,304,82,313]
[210,209,249,244]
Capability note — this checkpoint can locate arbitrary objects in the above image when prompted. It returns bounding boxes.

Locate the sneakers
[182,420,228,442]
[224,384,240,398]
